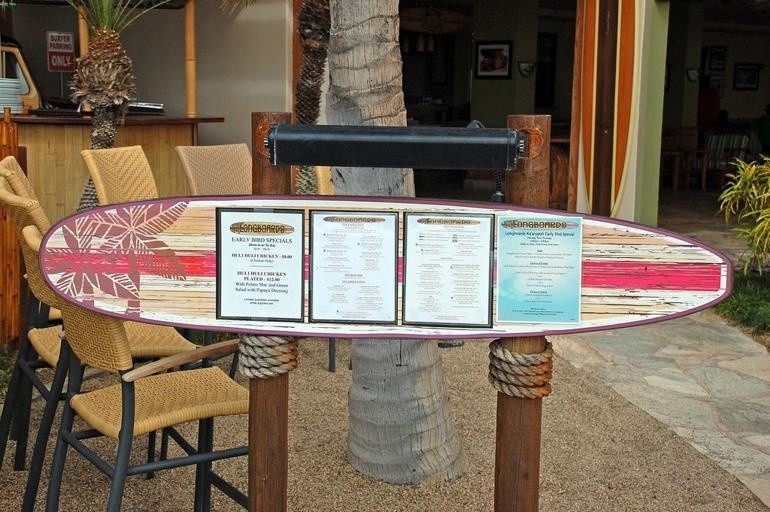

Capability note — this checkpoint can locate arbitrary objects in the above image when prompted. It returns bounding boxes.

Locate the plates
[0,77,24,118]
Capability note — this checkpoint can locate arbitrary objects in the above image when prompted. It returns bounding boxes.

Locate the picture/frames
[474,39,513,81]
[702,45,764,100]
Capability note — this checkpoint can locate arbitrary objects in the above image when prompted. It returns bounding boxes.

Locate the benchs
[748,128,769,169]
[682,133,749,191]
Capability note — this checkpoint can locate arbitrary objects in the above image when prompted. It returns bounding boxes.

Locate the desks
[662,149,713,193]
